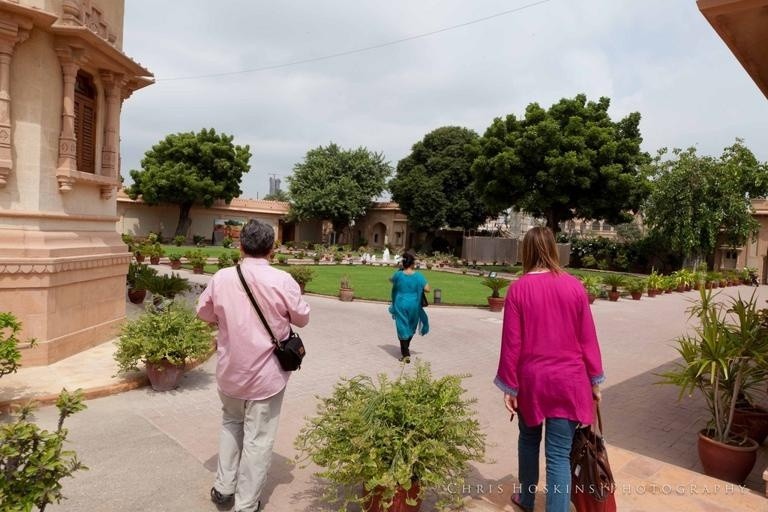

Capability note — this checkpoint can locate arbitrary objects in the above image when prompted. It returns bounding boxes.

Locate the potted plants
[287,354,490,511]
[700,308,767,444]
[576,265,759,303]
[649,279,759,485]
[123,235,508,311]
[112,299,212,392]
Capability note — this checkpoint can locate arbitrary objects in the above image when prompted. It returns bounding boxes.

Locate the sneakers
[210,487,260,512]
[399,357,411,364]
[511,493,534,512]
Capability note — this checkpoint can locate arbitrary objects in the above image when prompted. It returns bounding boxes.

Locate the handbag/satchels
[570,428,617,512]
[421,292,428,307]
[274,332,306,371]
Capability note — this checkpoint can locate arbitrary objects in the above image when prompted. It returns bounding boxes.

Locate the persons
[387,251,432,364]
[491,224,607,511]
[195,218,312,511]
